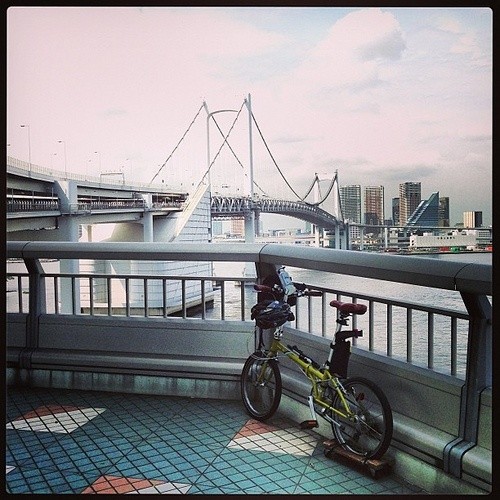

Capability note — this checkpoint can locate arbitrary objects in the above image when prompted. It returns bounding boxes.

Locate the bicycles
[234,264,395,461]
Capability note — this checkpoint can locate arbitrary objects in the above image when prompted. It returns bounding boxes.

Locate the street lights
[20,123,32,163]
[94,151,102,173]
[58,140,67,177]
